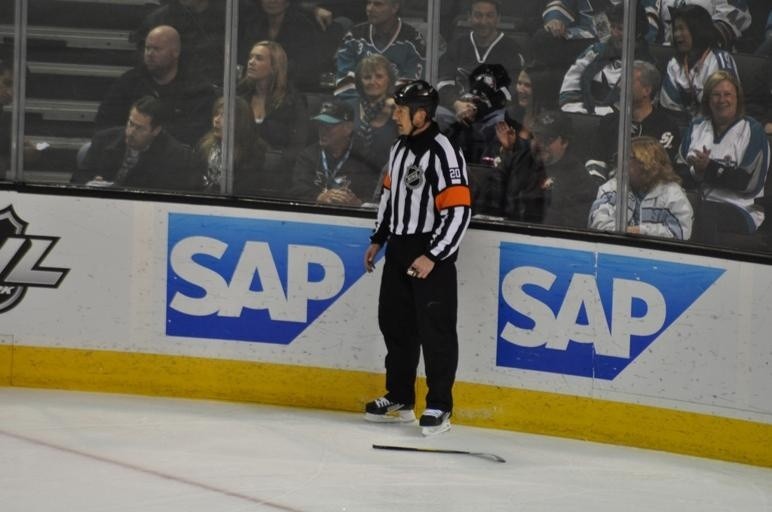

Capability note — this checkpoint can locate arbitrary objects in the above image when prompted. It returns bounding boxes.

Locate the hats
[528,109,567,137]
[309,99,355,126]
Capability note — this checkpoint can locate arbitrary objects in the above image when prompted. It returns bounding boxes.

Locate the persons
[74,1,770,255]
[363,81,472,428]
[1,57,49,178]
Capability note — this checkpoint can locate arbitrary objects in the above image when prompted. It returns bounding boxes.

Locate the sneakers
[364,394,416,423]
[419,409,452,436]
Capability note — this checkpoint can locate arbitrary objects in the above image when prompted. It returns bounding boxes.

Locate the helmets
[393,79,442,111]
